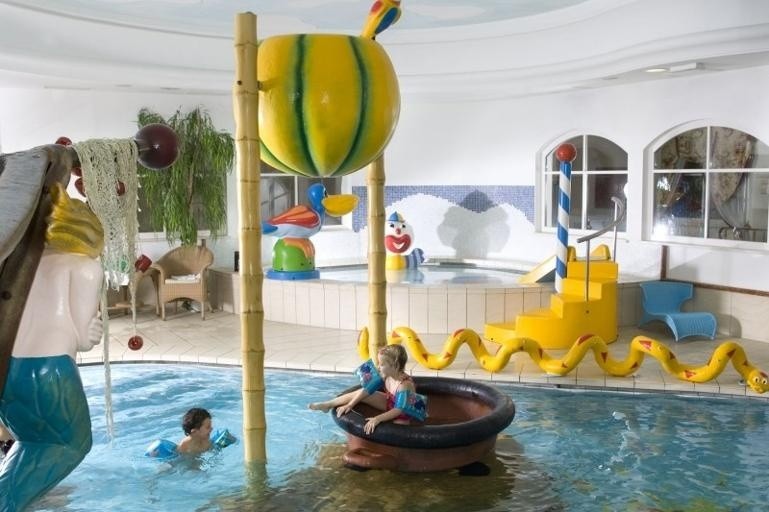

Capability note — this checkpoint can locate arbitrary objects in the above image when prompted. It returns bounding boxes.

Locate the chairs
[153,245,214,320]
[638,281,717,342]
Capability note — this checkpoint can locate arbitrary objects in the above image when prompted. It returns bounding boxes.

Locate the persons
[307,343,417,435]
[176,407,215,455]
[0,180,109,511]
